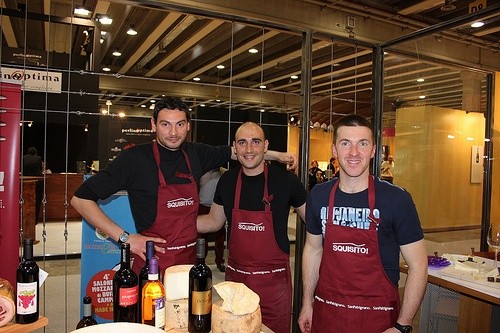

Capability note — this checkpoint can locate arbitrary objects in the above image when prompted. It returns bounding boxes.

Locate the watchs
[394,322,412,333]
[117,230,129,245]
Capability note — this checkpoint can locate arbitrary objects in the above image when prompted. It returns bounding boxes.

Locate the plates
[0,296,15,327]
[427,255,451,271]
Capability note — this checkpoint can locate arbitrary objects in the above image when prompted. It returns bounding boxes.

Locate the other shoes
[216,263,225,273]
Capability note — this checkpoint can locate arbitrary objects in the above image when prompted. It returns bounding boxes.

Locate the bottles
[76,296,97,330]
[141,257,167,330]
[112,243,140,324]
[14,238,39,324]
[188,238,211,333]
[138,241,162,324]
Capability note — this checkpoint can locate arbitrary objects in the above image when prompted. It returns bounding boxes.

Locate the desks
[39,172,84,223]
[0,315,48,333]
[399,251,500,333]
[20,176,48,247]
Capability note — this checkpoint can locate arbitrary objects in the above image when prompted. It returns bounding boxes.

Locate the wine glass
[487,224,500,270]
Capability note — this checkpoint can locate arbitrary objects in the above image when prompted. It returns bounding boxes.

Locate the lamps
[20,5,137,132]
[290,117,298,125]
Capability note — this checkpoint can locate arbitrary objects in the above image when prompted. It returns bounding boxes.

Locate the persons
[196,122,308,333]
[86,162,95,174]
[200,166,226,271]
[23,148,51,224]
[310,157,340,187]
[70,97,297,283]
[381,155,394,175]
[298,114,428,333]
